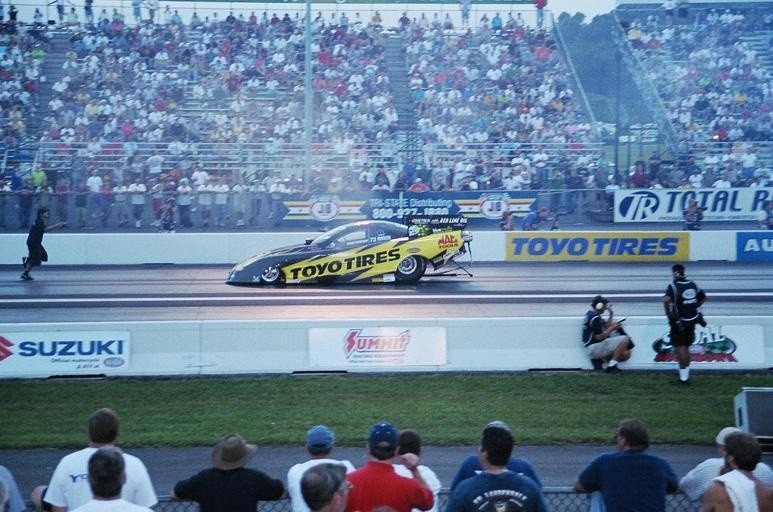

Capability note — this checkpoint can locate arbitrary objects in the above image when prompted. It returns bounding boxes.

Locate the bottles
[209,434,258,471]
[306,424,335,451]
[368,421,398,452]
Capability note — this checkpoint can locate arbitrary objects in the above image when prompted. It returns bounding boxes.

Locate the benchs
[0,20,772,210]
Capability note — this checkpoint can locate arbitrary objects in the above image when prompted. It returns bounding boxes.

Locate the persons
[704,430,772,512]
[1,1,307,228]
[442,425,548,512]
[618,2,772,189]
[344,421,435,512]
[0,464,68,512]
[169,432,283,512]
[285,424,356,510]
[684,198,707,229]
[311,2,631,231]
[520,207,553,229]
[448,420,544,492]
[572,416,680,510]
[298,460,356,512]
[18,206,48,280]
[67,447,156,512]
[659,263,709,386]
[757,199,772,229]
[579,296,634,376]
[677,425,772,502]
[500,210,514,229]
[393,430,442,512]
[42,406,159,512]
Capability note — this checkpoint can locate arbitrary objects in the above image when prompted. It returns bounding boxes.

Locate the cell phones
[695,312,706,327]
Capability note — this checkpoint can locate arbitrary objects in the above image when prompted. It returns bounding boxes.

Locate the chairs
[20,256,34,281]
[591,358,603,372]
[0,214,284,234]
[670,378,690,386]
[606,363,625,374]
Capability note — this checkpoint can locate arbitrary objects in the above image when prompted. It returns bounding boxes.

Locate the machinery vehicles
[221,212,480,290]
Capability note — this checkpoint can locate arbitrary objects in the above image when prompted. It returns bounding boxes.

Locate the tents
[595,297,604,310]
[673,271,688,278]
